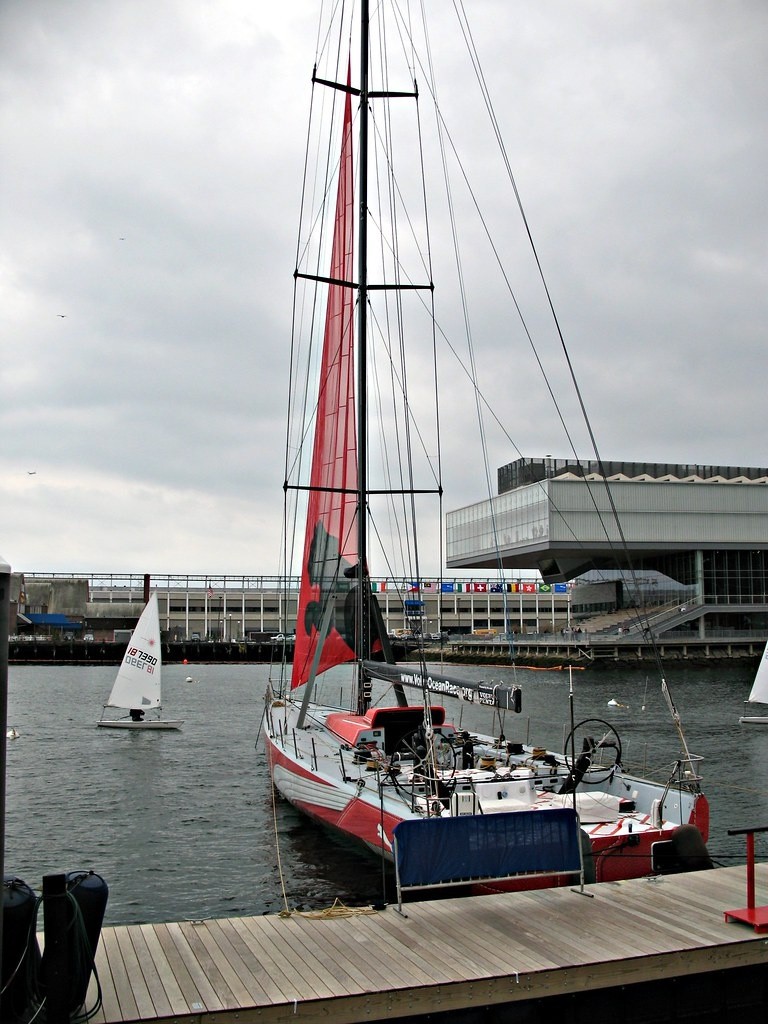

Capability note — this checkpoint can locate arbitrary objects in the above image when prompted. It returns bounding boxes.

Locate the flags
[507,583,519,592]
[474,584,486,592]
[523,584,535,593]
[539,583,551,592]
[555,584,566,592]
[372,582,385,592]
[19,577,26,604]
[424,582,437,593]
[408,582,419,591]
[458,584,470,592]
[442,583,453,592]
[490,584,503,593]
[208,587,213,597]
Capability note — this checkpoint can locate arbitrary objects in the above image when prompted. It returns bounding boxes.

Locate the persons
[678,606,685,612]
[130,709,145,721]
[617,622,630,635]
[544,627,551,641]
[561,627,582,642]
[506,629,517,639]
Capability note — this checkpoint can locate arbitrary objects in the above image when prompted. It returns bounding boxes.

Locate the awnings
[17,612,83,635]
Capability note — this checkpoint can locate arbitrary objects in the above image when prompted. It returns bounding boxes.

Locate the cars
[385,629,449,641]
[191,634,200,642]
[64,632,74,639]
[84,634,93,641]
[237,636,250,642]
[269,634,296,642]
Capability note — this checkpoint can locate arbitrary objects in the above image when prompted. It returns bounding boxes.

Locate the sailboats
[738,639,768,723]
[97,589,184,730]
[257,0,711,893]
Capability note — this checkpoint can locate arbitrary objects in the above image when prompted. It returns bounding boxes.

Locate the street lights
[228,614,232,643]
[237,621,240,642]
[220,621,224,643]
